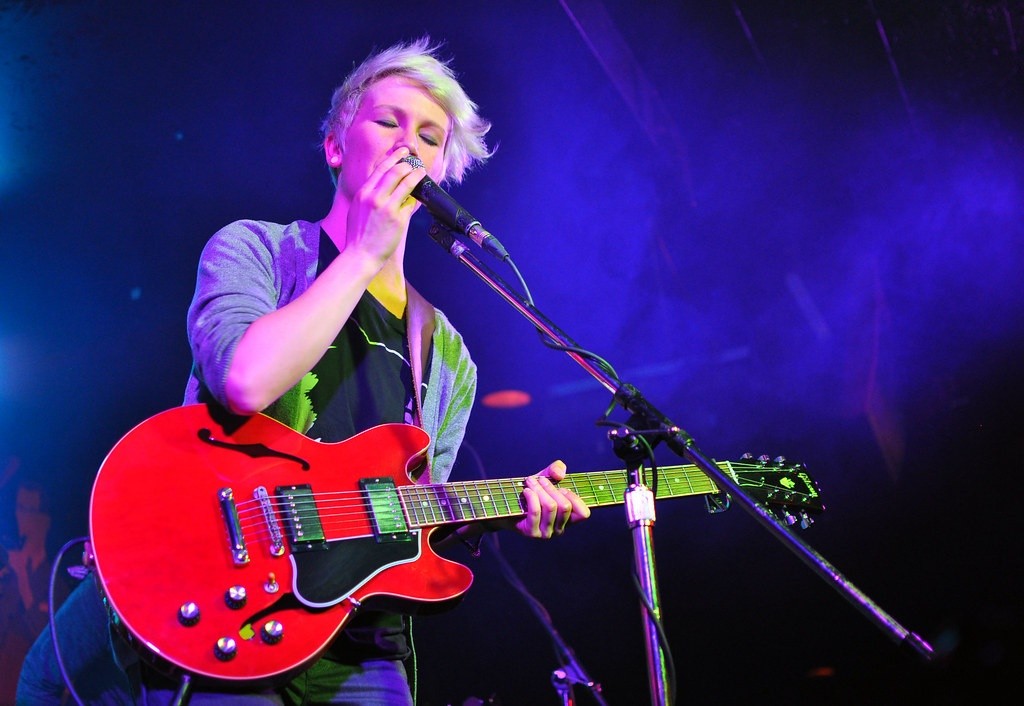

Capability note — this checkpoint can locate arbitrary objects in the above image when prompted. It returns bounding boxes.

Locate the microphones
[397,154,510,262]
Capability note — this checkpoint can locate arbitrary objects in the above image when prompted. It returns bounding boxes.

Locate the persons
[17,38,591,706]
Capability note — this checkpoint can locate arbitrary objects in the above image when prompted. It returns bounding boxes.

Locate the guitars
[86,402,832,694]
